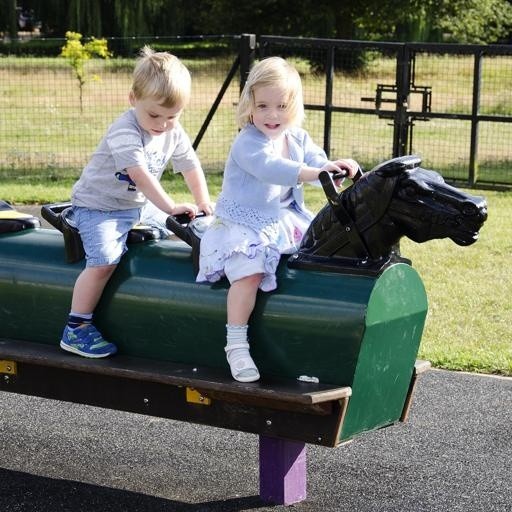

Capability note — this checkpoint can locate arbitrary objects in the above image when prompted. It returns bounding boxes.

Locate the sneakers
[59,323,116,360]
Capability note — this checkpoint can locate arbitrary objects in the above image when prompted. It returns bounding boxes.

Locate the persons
[59,52,216,359]
[196,55,359,383]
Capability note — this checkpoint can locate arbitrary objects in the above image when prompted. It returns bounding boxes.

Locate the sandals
[224,343,260,382]
